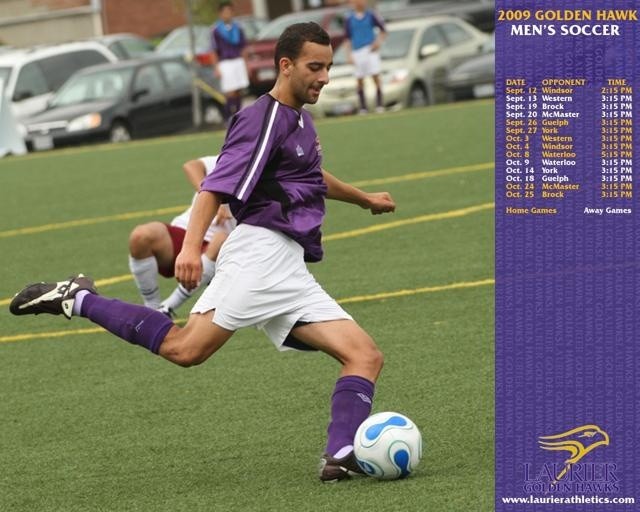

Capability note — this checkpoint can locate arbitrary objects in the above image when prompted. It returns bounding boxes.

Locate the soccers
[352,411,422,481]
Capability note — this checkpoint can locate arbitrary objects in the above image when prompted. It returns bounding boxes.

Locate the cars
[0,2,496,156]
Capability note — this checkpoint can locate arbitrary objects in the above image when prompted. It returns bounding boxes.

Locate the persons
[344,1,387,115]
[9,22,424,484]
[127,153,237,318]
[207,2,250,126]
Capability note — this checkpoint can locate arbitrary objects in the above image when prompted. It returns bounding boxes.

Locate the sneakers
[358,104,385,116]
[317,448,369,484]
[158,299,174,322]
[8,272,99,320]
